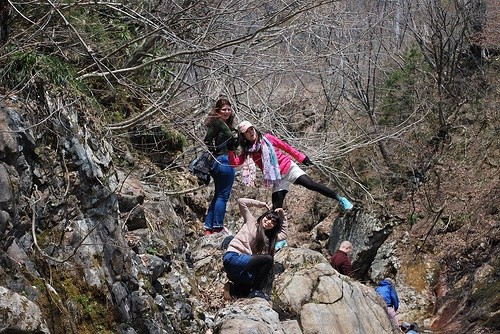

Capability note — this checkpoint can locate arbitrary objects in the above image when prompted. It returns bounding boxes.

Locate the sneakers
[205,228,213,236]
[247,288,270,300]
[275,239,287,253]
[230,279,238,299]
[214,227,232,235]
[337,194,353,211]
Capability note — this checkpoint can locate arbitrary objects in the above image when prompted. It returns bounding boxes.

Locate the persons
[330,240,353,279]
[204,99,240,236]
[228,120,353,253]
[375,276,400,329]
[222,197,288,301]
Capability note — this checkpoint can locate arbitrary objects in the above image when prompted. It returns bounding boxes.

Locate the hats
[237,120,253,134]
[401,322,410,331]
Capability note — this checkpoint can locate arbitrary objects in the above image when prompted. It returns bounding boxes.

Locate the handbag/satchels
[188,147,219,174]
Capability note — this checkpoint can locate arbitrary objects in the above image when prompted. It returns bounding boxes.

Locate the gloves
[227,139,235,151]
[302,156,315,166]
